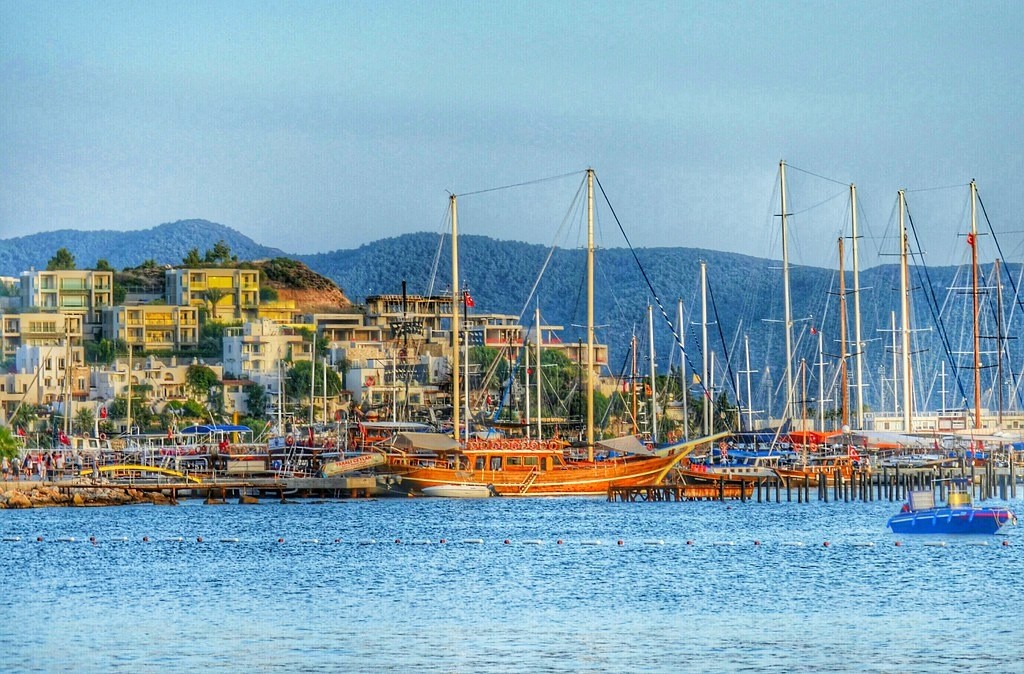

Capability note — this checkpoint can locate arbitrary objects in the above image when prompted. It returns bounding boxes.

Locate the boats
[420,483,501,497]
[885,478,1018,535]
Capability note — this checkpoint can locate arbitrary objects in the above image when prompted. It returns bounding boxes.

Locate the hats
[94,457,99,460]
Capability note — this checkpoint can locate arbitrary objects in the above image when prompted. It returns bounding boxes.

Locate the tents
[181,424,254,445]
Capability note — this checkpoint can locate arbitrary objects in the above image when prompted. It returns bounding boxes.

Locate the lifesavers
[287,436,294,445]
[795,443,800,452]
[83,431,90,440]
[810,443,818,452]
[100,432,107,441]
[466,441,558,451]
[195,446,202,454]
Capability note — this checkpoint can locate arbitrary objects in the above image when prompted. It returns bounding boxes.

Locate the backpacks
[13,458,19,469]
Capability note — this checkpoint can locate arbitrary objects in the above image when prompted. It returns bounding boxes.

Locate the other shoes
[11,479,19,481]
[3,478,8,482]
[39,478,46,481]
[23,478,31,481]
[90,482,97,485]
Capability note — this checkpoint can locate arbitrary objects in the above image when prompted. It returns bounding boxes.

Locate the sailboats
[66,159,1024,487]
[387,168,736,498]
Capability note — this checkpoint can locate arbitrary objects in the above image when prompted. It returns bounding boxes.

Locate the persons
[337,448,344,462]
[1007,441,1015,463]
[0,451,101,488]
[863,456,872,472]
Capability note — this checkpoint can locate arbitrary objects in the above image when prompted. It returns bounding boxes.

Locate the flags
[862,433,868,450]
[970,440,985,455]
[809,431,821,445]
[486,390,492,407]
[307,427,315,446]
[932,428,941,451]
[464,288,474,308]
[167,425,174,441]
[57,427,71,446]
[17,425,26,436]
[847,445,860,461]
